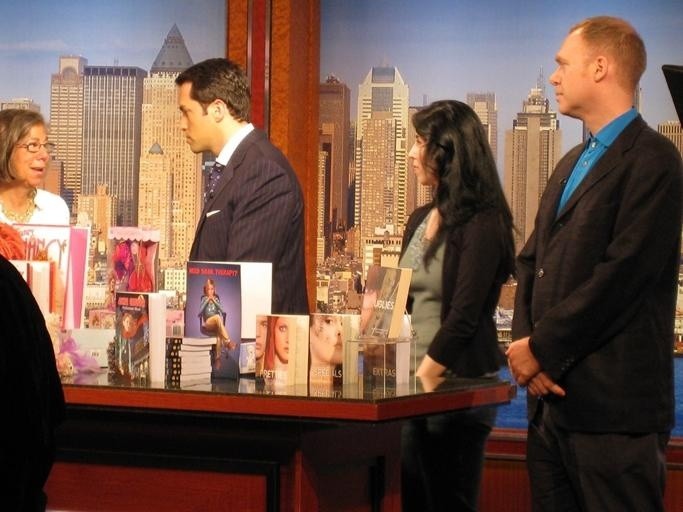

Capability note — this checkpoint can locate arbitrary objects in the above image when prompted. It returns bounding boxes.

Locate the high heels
[225,341,237,350]
[214,357,221,371]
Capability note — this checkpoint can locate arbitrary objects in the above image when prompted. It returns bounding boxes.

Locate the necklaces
[2,189,38,224]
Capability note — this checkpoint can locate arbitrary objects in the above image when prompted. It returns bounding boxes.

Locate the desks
[42,366,518,511]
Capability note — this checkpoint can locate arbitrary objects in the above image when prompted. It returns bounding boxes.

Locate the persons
[504,15,683,512]
[397,101,521,511]
[0,254,63,512]
[0,109,71,311]
[174,58,309,313]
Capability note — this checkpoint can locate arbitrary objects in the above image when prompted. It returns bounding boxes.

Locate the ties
[202,162,224,207]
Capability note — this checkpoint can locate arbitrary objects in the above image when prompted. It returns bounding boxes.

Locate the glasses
[14,143,54,153]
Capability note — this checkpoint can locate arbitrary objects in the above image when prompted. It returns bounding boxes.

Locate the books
[89,225,413,389]
